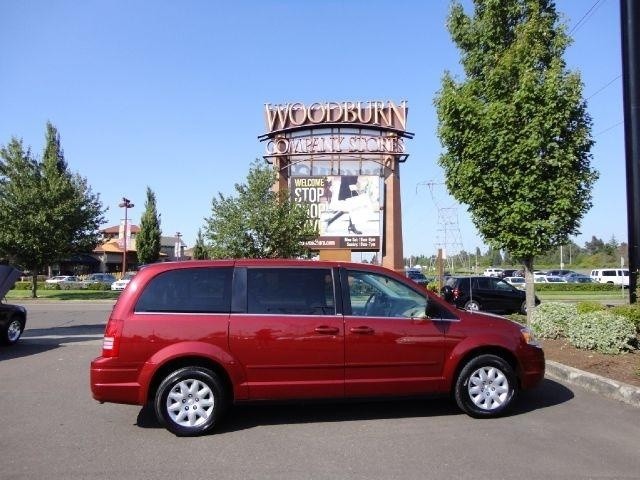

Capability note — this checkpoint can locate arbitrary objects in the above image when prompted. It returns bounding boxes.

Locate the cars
[403,267,632,316]
[42,271,139,294]
[0,263,27,347]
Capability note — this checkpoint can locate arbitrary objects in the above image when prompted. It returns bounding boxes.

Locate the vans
[89,258,546,438]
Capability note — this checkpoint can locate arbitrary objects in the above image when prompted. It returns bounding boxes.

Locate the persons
[323,175,364,235]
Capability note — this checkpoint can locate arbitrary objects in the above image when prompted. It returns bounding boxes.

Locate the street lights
[118,197,135,275]
[174,231,182,262]
